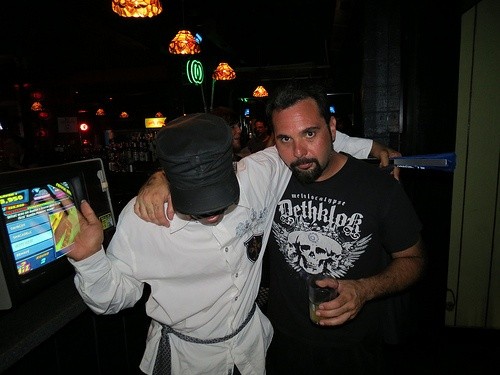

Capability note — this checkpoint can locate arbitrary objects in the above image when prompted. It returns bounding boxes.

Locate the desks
[0,270,129,375]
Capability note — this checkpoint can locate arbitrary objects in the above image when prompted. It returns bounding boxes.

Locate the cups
[306,275,339,324]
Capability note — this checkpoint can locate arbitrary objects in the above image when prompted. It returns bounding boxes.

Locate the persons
[211,107,242,163]
[137,85,425,375]
[254,119,275,148]
[18,109,402,375]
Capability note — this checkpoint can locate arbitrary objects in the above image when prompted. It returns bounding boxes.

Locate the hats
[156,113,240,215]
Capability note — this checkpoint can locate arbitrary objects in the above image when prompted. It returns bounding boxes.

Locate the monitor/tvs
[0,156,117,289]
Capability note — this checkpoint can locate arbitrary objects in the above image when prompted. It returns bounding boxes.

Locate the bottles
[54,136,158,173]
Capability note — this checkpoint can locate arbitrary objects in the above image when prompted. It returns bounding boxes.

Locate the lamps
[30,100,44,112]
[168,0,203,56]
[251,68,269,99]
[78,105,88,113]
[211,55,238,81]
[96,104,106,117]
[111,0,163,19]
